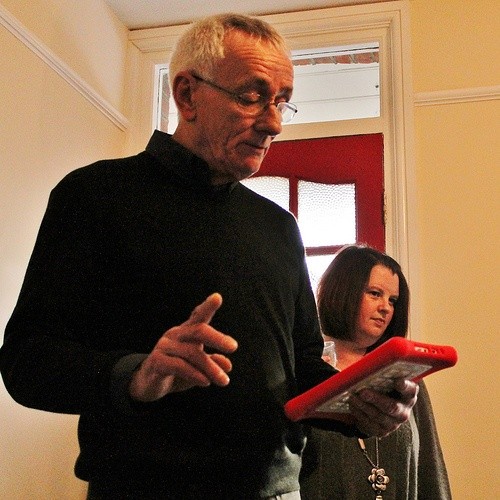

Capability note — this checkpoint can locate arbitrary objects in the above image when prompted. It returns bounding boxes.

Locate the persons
[1,12,420,500]
[298,242,454,500]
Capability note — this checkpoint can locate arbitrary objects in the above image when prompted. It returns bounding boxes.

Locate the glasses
[195,72,299,123]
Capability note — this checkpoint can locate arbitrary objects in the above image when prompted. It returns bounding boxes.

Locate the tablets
[284,337,457,425]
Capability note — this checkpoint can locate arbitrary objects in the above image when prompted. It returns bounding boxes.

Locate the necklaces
[356,433,390,500]
